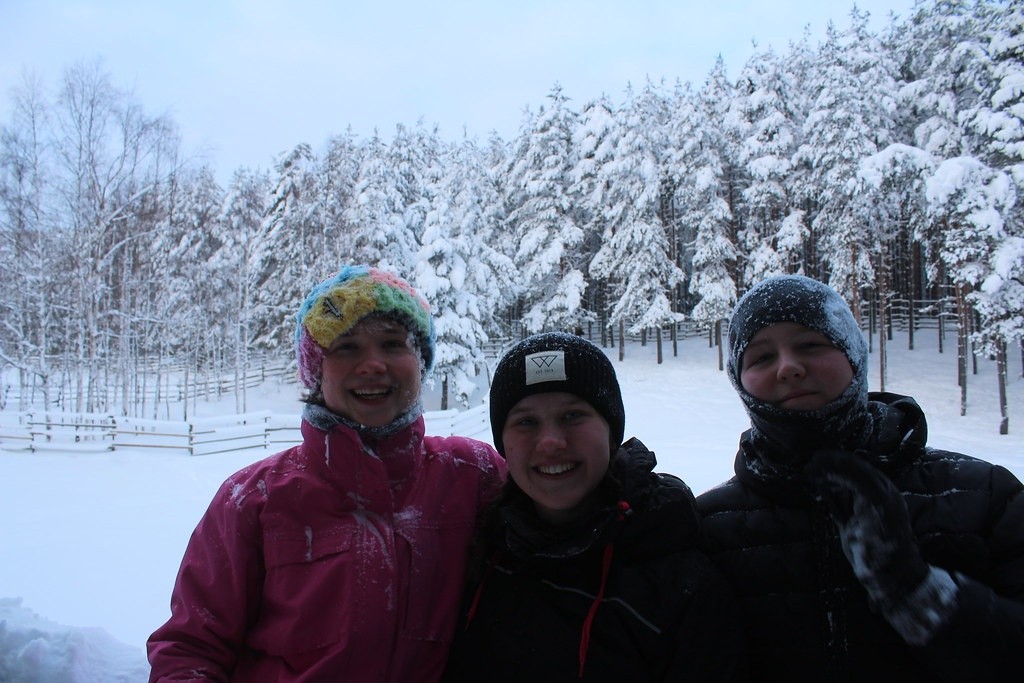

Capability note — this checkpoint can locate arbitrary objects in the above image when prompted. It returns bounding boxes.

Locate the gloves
[812,453,932,616]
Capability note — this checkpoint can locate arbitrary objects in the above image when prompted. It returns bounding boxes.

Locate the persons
[145,266,507,683]
[440,331,736,683]
[694,275,1024,683]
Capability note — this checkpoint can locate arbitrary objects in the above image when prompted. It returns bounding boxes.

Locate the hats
[729,275,863,383]
[490,331,626,458]
[295,264,435,389]
